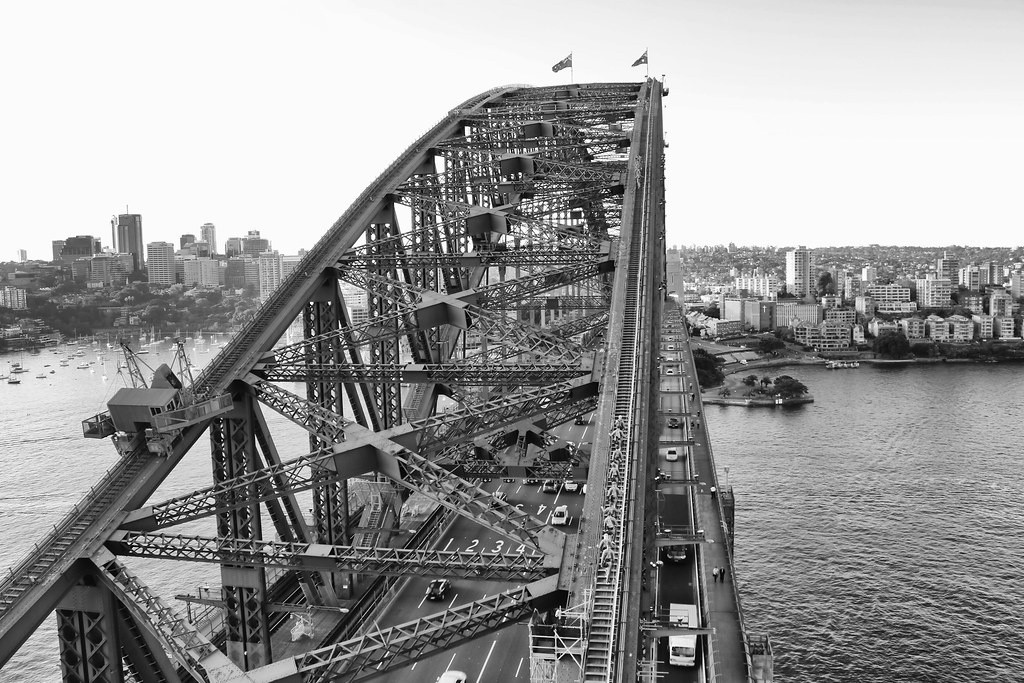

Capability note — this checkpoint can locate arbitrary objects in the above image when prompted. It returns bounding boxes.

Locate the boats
[825,362,860,369]
[1,330,229,385]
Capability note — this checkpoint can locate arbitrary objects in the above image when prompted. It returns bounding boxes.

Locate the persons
[598,415,627,582]
[634,154,645,189]
[712,566,726,583]
[689,383,717,499]
[642,518,647,661]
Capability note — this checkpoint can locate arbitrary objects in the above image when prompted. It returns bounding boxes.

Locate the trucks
[669,603,698,667]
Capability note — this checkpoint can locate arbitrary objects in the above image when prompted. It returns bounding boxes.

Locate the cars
[435,669,467,683]
[522,477,587,494]
[551,504,569,526]
[489,492,507,510]
[424,579,451,602]
[575,415,584,426]
[665,447,678,462]
[667,311,675,376]
[668,540,687,563]
[668,417,679,429]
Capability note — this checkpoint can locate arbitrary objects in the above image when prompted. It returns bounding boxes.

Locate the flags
[553,54,573,72]
[632,51,648,67]
